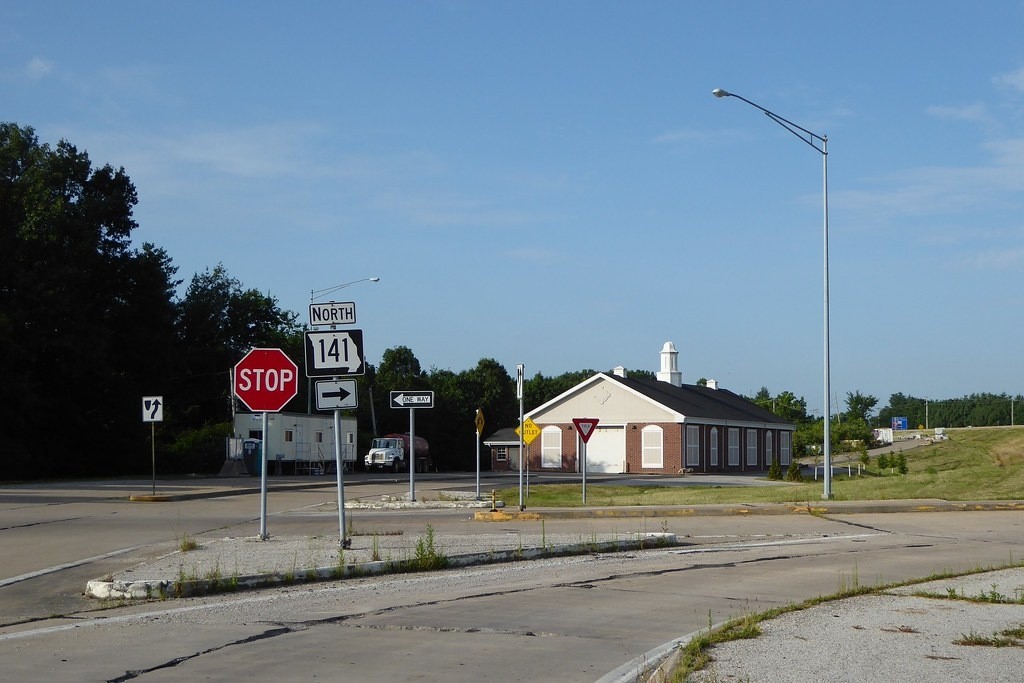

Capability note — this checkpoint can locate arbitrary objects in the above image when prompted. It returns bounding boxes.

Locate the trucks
[872,428,894,445]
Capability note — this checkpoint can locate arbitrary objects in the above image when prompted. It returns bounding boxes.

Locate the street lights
[307,278,380,415]
[712,86,835,501]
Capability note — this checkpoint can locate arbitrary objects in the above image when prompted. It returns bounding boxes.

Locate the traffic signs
[390,390,435,409]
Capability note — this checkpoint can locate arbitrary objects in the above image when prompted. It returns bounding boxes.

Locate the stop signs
[235,348,299,413]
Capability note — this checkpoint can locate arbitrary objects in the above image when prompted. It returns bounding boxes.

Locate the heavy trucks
[364,433,432,472]
